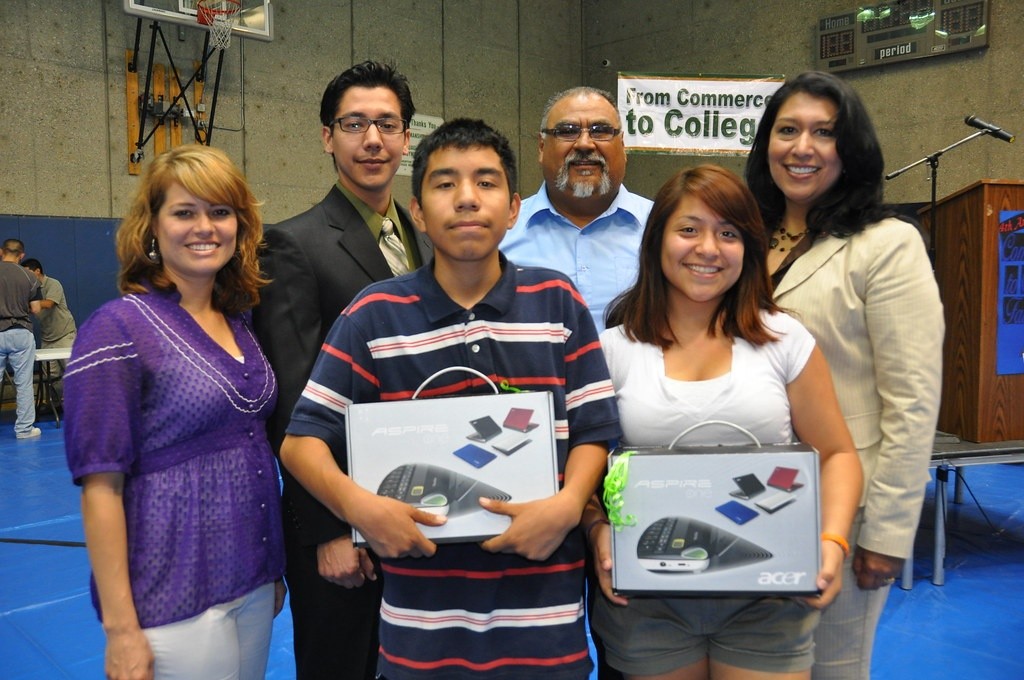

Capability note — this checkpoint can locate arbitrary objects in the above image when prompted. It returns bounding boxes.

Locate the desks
[34,347,72,428]
[900,430,1024,588]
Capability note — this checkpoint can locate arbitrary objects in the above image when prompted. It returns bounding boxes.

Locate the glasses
[542,122,620,141]
[328,116,406,134]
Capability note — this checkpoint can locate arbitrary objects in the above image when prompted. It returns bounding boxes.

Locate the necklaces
[774,224,810,241]
[769,238,795,251]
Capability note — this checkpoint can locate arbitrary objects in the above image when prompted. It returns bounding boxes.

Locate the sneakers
[17,426,41,439]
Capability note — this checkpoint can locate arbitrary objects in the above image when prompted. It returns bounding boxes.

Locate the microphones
[962,114,1018,143]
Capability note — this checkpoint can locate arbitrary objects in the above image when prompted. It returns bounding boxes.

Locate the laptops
[452,407,540,469]
[635,516,710,573]
[715,463,805,525]
[375,463,450,518]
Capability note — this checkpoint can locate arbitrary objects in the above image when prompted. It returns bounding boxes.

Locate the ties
[379,219,409,277]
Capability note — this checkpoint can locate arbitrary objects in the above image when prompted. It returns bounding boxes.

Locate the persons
[62,144,287,680]
[257,62,946,680]
[20,258,77,417]
[0,238,43,439]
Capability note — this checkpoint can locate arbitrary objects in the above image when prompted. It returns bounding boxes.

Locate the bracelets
[585,519,610,545]
[822,532,849,560]
